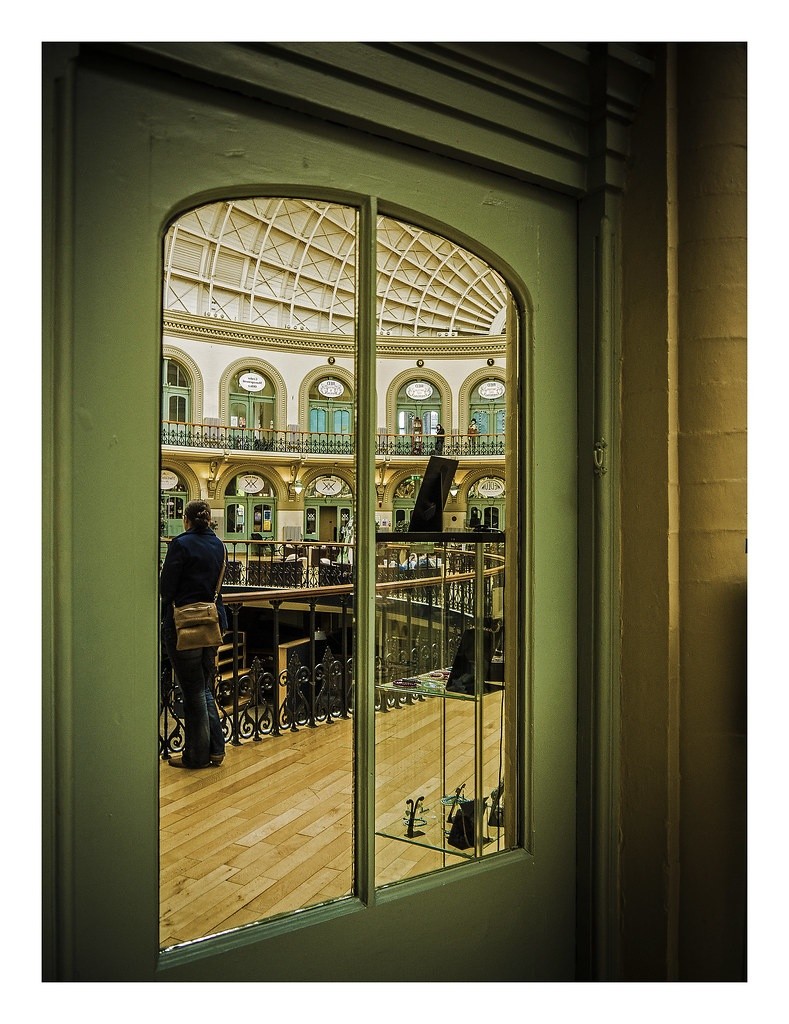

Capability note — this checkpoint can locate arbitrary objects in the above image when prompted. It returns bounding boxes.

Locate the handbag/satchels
[172,601,224,651]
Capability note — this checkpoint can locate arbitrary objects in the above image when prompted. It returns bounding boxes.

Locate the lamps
[294,466,303,495]
[449,479,459,498]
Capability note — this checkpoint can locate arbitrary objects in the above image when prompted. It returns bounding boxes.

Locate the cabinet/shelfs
[212,630,255,718]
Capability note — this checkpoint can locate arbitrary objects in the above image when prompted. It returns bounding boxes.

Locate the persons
[468,419,478,454]
[160,499,230,769]
[434,424,445,455]
[414,553,435,568]
[403,553,417,570]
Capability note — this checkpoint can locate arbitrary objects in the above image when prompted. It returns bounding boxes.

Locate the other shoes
[168,754,226,768]
